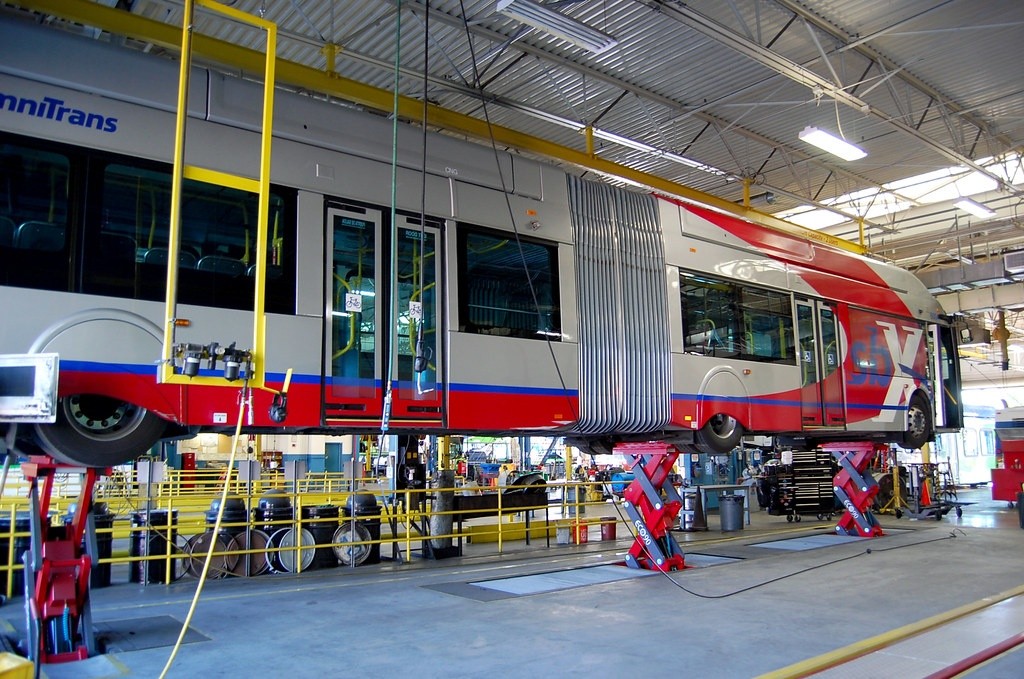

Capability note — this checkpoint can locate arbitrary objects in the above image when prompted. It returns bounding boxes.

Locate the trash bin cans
[129,509,177,585]
[300,504,339,569]
[600,517,618,541]
[0,513,52,597]
[342,488,382,565]
[555,524,570,545]
[206,491,247,539]
[253,489,294,572]
[64,502,115,586]
[719,495,745,532]
[573,521,589,543]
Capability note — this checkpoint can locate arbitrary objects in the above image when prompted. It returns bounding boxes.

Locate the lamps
[798,124,868,162]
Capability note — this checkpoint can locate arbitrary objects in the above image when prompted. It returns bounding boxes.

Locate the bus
[0,15,972,468]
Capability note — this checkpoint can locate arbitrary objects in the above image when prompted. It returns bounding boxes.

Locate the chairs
[0,216,295,284]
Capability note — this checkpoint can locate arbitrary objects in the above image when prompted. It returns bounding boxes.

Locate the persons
[576,465,584,475]
[538,464,543,470]
[588,464,599,482]
